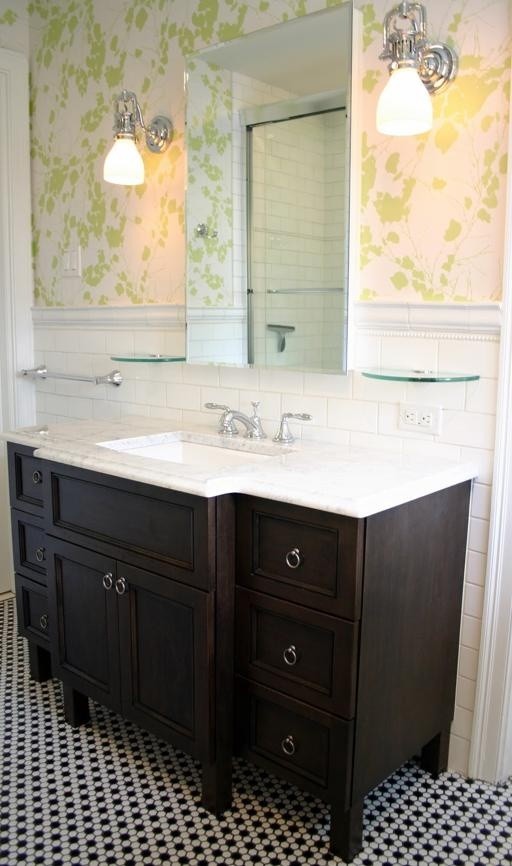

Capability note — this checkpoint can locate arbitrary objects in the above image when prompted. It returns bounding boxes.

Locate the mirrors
[181,1,363,377]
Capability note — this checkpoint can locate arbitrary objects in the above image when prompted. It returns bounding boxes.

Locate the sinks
[96,430,298,468]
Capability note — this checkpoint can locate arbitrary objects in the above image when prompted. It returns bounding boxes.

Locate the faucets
[218,399,265,438]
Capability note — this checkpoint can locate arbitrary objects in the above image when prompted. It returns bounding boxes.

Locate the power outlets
[396,404,443,436]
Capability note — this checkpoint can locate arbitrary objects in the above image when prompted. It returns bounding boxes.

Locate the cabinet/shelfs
[231,482,475,804]
[45,465,240,765]
[8,441,58,652]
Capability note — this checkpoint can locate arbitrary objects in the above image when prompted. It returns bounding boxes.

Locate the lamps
[101,89,174,188]
[374,2,457,137]
